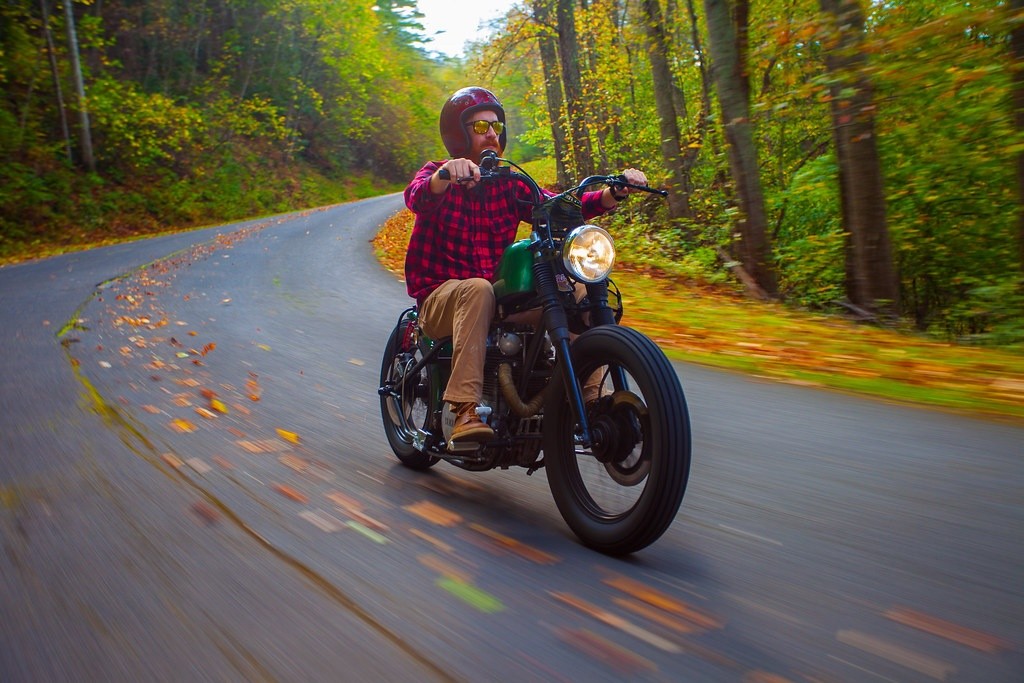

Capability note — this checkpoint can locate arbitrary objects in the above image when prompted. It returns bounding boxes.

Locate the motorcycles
[378,149,693,555]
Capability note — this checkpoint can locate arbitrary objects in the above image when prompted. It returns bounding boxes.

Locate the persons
[404,86,649,452]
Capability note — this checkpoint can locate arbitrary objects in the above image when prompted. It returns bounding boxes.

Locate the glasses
[463,119,505,134]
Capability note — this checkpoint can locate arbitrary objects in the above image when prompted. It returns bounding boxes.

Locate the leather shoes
[450,402,494,441]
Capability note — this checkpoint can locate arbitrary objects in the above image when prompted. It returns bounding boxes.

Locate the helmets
[439,86,507,157]
